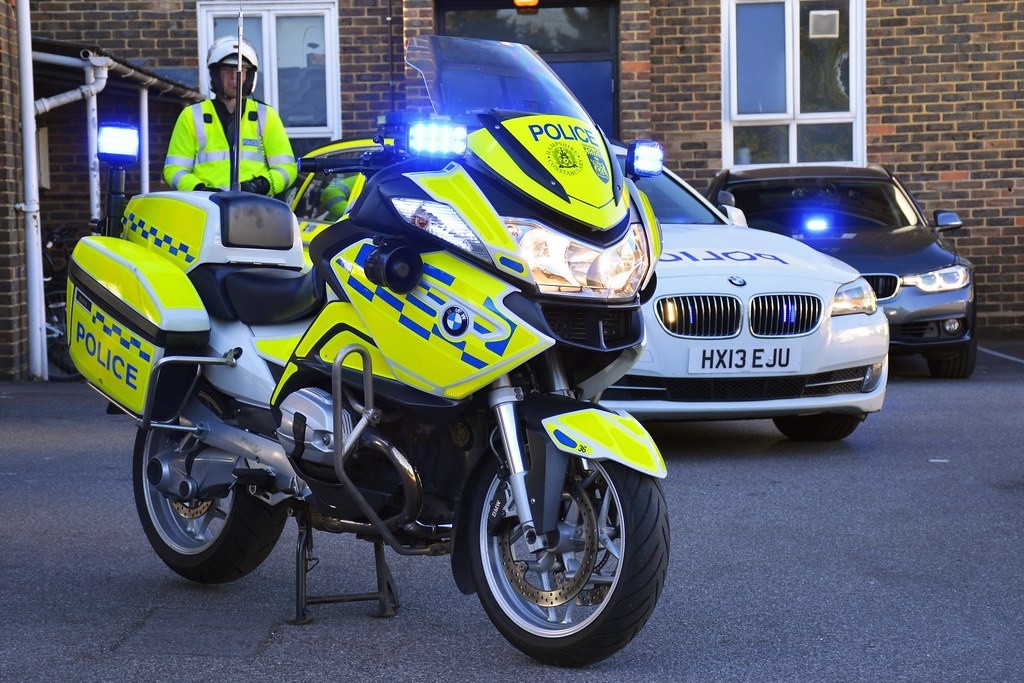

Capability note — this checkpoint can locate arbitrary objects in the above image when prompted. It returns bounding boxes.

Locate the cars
[701,160,977,381]
[585,139,890,443]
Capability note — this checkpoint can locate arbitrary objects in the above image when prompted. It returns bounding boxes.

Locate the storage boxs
[62,237,209,418]
[123,193,302,274]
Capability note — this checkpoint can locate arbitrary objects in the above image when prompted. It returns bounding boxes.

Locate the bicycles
[44,224,86,383]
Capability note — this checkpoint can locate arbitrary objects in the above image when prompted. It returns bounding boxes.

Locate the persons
[163,35,298,197]
[320,174,368,222]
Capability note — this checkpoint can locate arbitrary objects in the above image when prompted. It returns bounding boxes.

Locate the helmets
[207,37,259,97]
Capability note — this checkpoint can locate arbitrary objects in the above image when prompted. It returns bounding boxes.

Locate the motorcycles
[65,35,672,670]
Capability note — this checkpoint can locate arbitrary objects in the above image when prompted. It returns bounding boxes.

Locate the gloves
[240,175,270,195]
[193,183,223,192]
